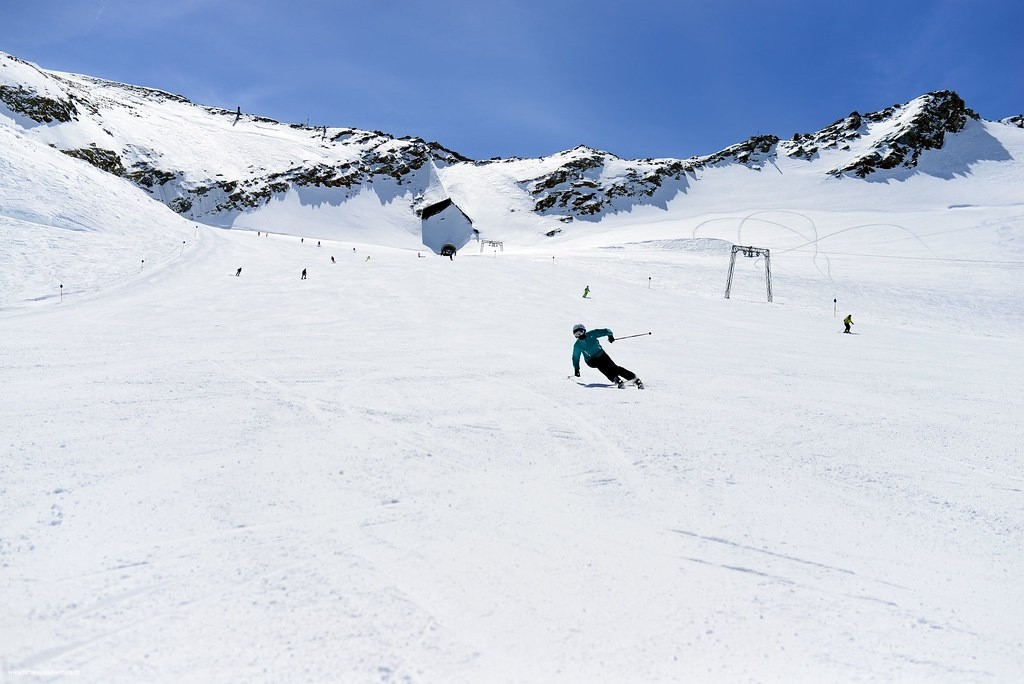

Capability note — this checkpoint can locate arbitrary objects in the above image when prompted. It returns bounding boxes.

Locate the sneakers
[634,378,644,389]
[614,377,625,389]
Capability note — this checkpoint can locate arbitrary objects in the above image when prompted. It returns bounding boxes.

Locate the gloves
[608,335,614,343]
[575,368,580,377]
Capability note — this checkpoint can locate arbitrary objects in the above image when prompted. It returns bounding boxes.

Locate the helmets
[573,323,586,338]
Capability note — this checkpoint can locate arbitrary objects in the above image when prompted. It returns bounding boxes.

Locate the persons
[301,269,308,280]
[843,315,854,333]
[236,267,243,276]
[582,286,590,298]
[571,324,644,390]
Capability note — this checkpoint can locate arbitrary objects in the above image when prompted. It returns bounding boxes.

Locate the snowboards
[578,376,640,388]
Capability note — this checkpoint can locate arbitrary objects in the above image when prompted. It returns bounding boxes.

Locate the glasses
[574,330,584,337]
